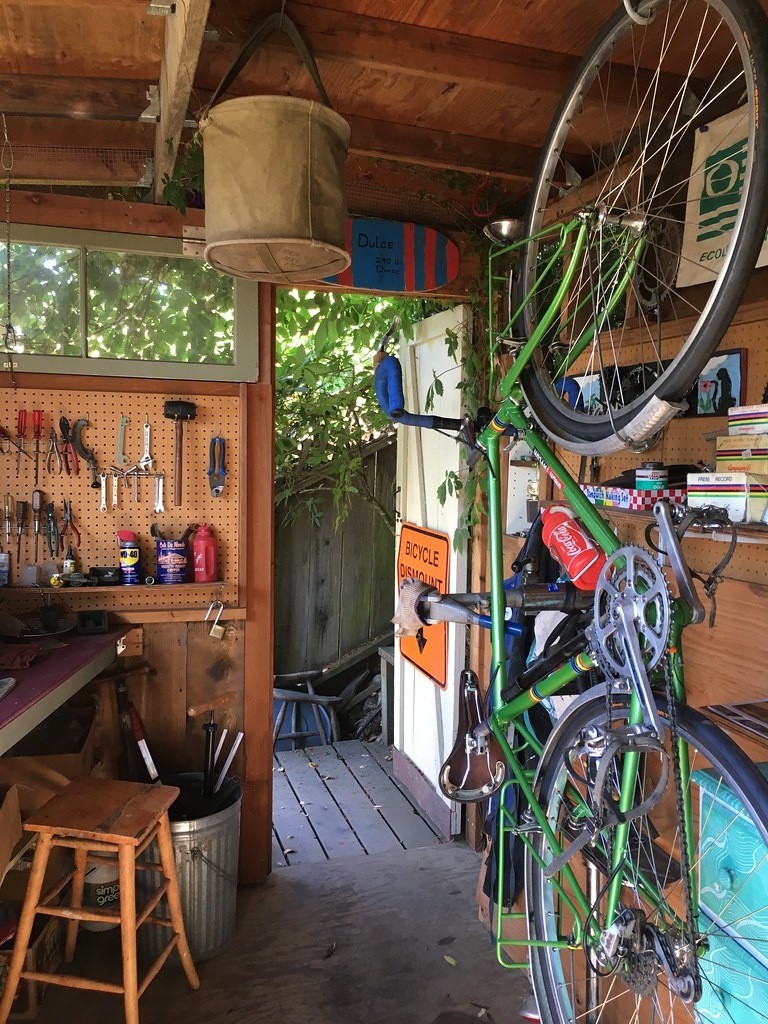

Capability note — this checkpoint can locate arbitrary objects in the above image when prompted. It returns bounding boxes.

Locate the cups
[156,539,188,585]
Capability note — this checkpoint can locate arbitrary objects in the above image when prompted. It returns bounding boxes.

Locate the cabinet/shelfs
[475,153,768,1024]
[690,762,768,1024]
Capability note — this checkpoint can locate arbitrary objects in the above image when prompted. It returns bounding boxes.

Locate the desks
[0,623,136,772]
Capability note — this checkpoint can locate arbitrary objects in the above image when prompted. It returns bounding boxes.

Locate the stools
[0,777,200,1024]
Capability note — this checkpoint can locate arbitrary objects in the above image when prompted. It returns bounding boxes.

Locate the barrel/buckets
[136,771,245,966]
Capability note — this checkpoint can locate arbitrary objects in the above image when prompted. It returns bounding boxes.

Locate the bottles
[192,523,217,583]
[541,502,612,591]
[81,849,121,932]
[635,462,669,490]
[63,544,76,574]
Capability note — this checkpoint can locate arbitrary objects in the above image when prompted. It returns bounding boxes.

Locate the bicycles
[366,1,768,1024]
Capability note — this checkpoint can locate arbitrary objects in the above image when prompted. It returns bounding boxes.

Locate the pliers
[46,429,62,475]
[207,438,227,497]
[59,416,80,475]
[60,498,81,551]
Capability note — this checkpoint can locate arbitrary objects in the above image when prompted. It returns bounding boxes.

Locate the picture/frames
[569,347,749,418]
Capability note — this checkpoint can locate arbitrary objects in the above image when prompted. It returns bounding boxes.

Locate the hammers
[164,401,196,506]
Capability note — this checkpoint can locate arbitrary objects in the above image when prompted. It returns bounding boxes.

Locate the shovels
[45,503,60,557]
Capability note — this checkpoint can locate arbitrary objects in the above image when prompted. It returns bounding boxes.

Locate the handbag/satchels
[197,13,351,284]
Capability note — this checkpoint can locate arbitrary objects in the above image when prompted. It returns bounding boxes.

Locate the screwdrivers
[17,410,26,474]
[31,490,42,562]
[33,410,42,483]
[15,501,26,564]
[3,495,13,544]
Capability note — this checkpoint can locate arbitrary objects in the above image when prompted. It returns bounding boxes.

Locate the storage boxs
[687,473,768,524]
[0,705,115,905]
[716,461,768,485]
[577,483,687,511]
[716,436,768,449]
[0,890,72,1021]
